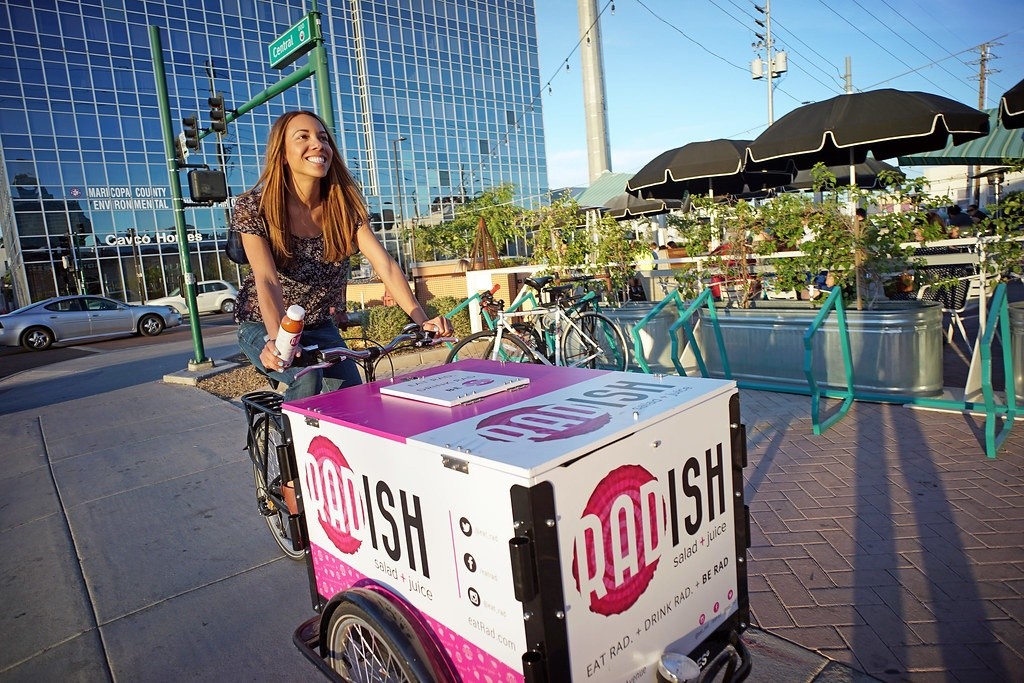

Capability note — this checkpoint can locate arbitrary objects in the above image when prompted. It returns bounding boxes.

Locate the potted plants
[665,160,961,398]
[956,191,1024,407]
[526,188,684,375]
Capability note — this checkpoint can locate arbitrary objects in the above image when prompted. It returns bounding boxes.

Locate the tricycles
[240,323,752,683]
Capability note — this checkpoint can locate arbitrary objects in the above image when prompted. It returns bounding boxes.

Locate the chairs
[718,280,752,302]
[760,279,798,301]
[890,245,976,367]
[950,273,1003,354]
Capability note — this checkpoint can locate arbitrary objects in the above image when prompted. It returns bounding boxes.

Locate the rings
[449,327,453,330]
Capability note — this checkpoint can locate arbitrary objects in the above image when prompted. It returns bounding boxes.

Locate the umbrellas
[575,192,685,246]
[773,157,905,211]
[682,184,785,211]
[742,88,990,307]
[626,138,798,249]
[997,77,1024,130]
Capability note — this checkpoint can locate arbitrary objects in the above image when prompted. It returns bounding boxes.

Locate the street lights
[392,137,409,280]
[970,167,1013,220]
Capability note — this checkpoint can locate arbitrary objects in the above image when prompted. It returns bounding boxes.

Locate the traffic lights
[173,138,185,165]
[183,116,200,152]
[207,96,228,135]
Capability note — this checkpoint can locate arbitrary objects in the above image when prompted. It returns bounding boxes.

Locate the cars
[145,279,239,317]
[0,294,183,352]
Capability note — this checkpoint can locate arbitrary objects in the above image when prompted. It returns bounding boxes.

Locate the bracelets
[421,319,429,325]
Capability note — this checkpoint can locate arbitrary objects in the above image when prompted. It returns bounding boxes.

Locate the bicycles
[442,273,629,373]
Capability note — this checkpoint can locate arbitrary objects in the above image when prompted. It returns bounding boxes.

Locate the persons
[708,212,851,301]
[648,241,678,250]
[234,111,453,513]
[855,208,878,233]
[883,204,993,309]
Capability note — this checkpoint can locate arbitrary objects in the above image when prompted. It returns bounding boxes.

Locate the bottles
[275,305,305,368]
